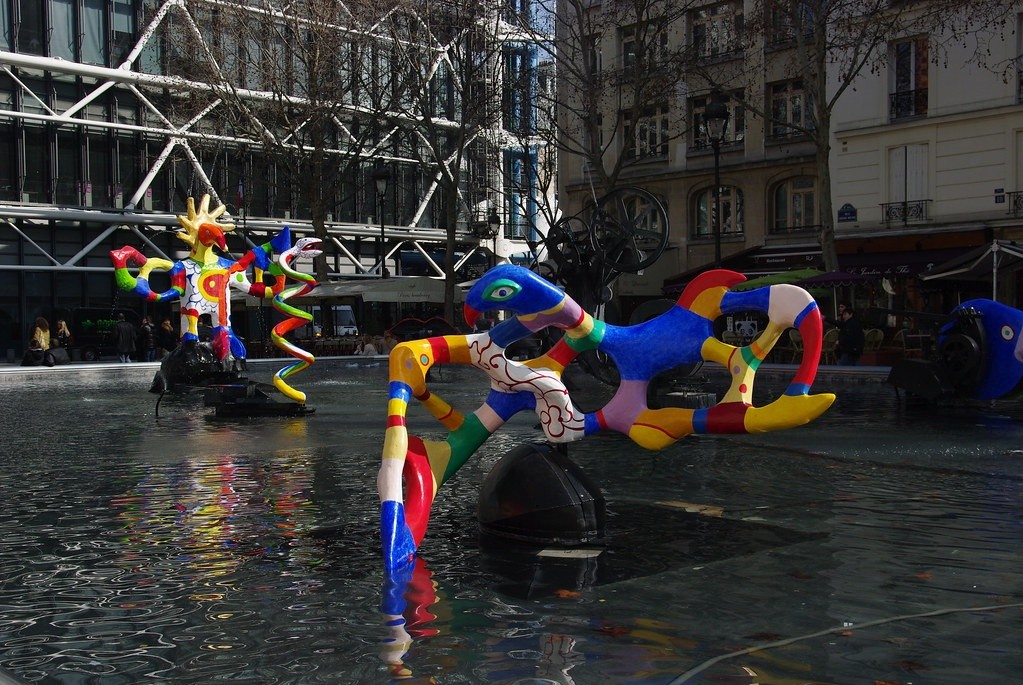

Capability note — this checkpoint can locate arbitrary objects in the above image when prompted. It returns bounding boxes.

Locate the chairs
[821,327,840,365]
[722,330,765,347]
[865,328,883,350]
[889,329,910,360]
[789,329,804,365]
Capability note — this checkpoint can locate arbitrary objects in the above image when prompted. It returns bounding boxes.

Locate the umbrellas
[789,272,866,321]
[736,268,825,290]
[918,239,1023,302]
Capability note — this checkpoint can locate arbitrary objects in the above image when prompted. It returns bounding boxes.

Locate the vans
[292,306,360,341]
[46,307,159,362]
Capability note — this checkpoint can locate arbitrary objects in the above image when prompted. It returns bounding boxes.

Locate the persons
[44,338,70,367]
[158,319,177,360]
[198,316,214,342]
[20,340,45,366]
[832,301,849,329]
[139,316,156,362]
[834,309,865,366]
[30,317,50,352]
[113,313,137,363]
[55,320,70,355]
[354,333,398,355]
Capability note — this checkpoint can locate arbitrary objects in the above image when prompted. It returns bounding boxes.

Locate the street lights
[487,206,502,327]
[369,156,392,329]
[699,88,733,261]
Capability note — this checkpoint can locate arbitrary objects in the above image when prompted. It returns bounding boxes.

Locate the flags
[236,180,243,210]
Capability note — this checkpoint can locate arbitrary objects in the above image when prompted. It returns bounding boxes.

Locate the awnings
[662,244,978,296]
